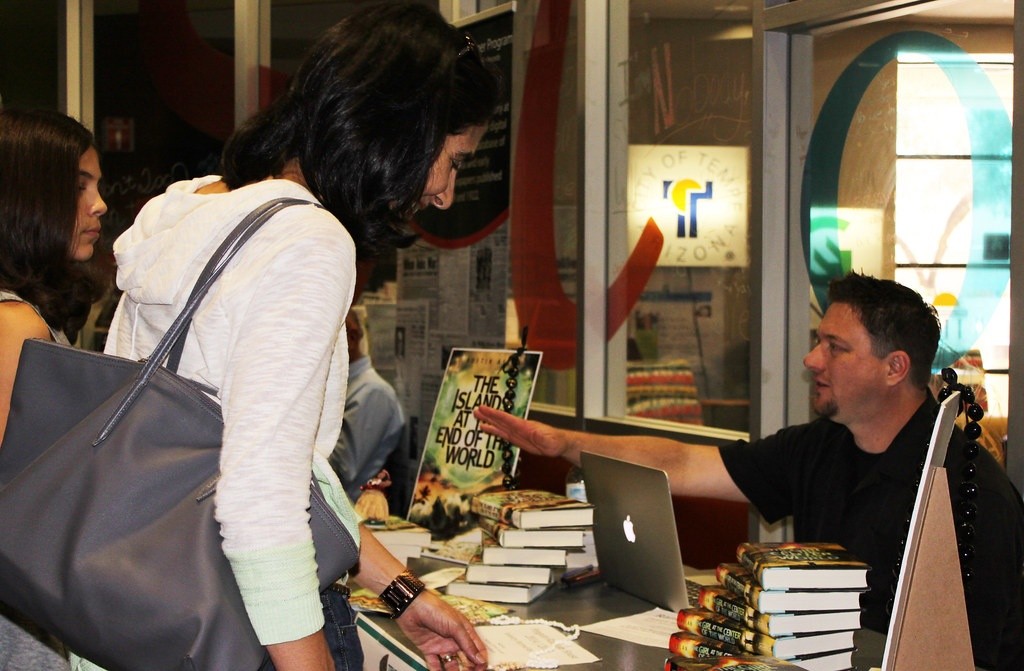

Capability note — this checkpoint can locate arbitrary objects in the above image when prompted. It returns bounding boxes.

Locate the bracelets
[377,568,425,621]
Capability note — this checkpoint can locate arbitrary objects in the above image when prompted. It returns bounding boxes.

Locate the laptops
[578,449,724,613]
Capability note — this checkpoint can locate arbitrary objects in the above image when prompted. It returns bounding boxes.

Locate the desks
[346,522,888,671]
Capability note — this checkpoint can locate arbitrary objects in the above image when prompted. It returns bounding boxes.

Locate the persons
[0,105,107,670]
[68,0,491,670]
[471,267,1024,671]
[326,307,406,512]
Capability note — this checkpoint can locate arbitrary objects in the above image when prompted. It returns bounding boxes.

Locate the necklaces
[499,325,522,491]
[886,367,984,621]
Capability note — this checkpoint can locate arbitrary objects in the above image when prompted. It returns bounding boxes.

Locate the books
[664,541,872,671]
[444,489,597,605]
[345,514,431,582]
